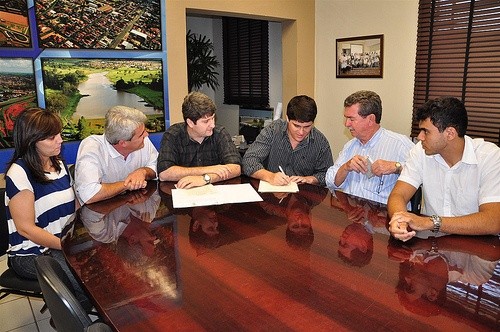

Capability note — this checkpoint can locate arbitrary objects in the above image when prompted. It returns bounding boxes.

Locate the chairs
[35,255,114,332]
[0,187,48,312]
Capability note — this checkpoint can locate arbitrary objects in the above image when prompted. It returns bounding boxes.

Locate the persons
[387,233,500,316]
[329,188,390,267]
[157,91,241,189]
[79,183,161,269]
[387,96,500,242]
[4,106,94,330]
[74,105,159,204]
[242,96,334,187]
[339,49,380,74]
[161,191,235,251]
[251,177,328,249]
[325,91,416,212]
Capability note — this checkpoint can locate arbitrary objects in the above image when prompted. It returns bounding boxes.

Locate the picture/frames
[336,35,384,78]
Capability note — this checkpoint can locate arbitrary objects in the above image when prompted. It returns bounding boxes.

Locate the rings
[188,184,192,187]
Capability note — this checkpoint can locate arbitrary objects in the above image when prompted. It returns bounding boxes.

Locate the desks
[61,173,500,332]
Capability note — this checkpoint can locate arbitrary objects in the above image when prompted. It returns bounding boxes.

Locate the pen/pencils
[278,165,285,175]
[367,154,374,164]
[279,198,283,204]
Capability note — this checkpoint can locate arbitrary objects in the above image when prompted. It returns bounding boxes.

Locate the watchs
[202,174,211,185]
[395,161,401,174]
[430,215,442,234]
